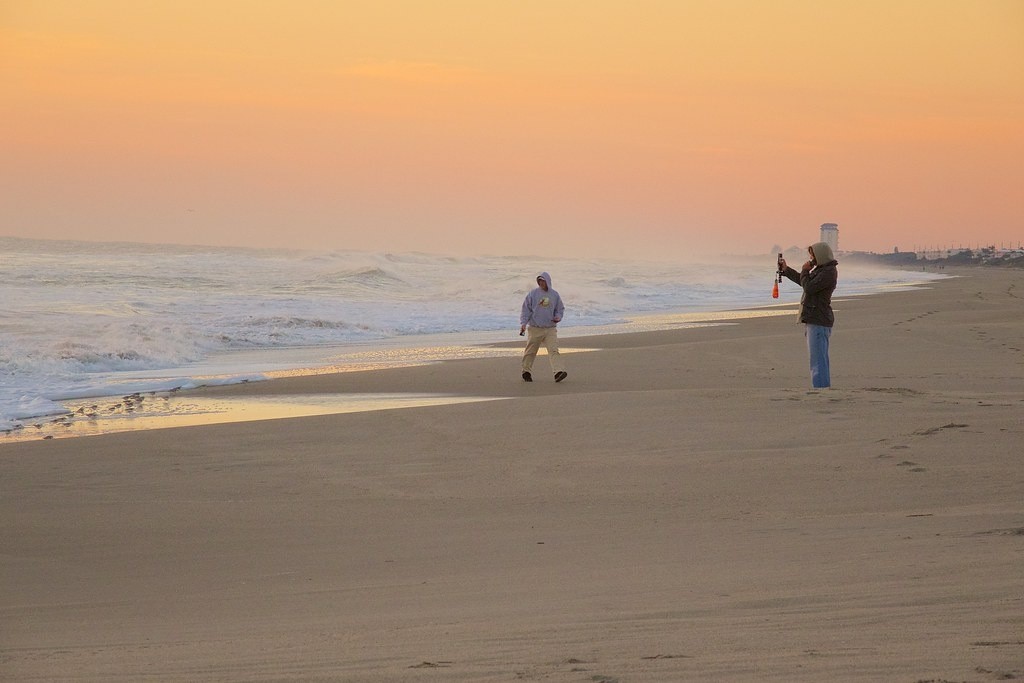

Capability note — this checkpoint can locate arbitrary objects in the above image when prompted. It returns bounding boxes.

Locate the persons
[780,242,838,387]
[520,272,567,382]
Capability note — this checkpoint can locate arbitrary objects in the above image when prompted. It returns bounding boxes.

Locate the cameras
[778,254,783,266]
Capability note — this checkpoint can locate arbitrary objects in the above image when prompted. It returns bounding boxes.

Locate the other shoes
[555,371,567,382]
[521,372,532,383]
